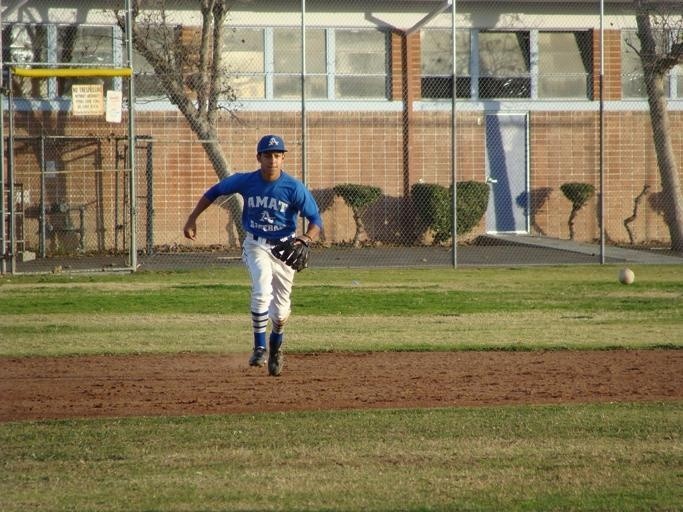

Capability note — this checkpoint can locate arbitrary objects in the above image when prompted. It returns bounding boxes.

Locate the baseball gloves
[271,234,311,273]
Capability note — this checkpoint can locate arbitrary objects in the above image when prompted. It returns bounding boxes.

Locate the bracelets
[303,233,312,241]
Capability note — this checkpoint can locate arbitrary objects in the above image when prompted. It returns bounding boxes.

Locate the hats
[257,135,288,153]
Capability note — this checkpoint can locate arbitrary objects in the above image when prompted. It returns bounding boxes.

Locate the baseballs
[619,267,634,285]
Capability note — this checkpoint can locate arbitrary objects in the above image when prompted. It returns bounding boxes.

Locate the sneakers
[249,347,267,367]
[269,344,284,375]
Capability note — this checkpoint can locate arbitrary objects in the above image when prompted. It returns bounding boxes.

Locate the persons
[183,135,322,376]
